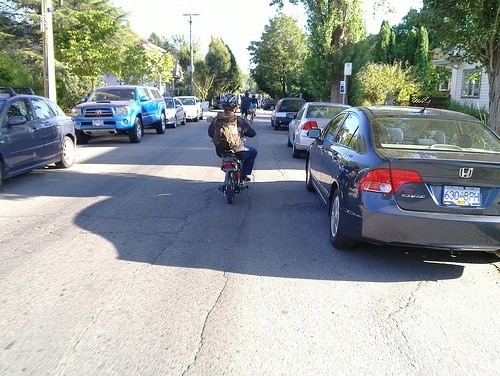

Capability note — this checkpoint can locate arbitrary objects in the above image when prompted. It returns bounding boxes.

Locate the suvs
[71,85,167,143]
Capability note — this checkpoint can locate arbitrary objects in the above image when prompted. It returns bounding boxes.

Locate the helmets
[222,93,237,107]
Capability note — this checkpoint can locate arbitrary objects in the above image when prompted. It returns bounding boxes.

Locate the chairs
[431,129,446,144]
[379,128,404,144]
[97,94,106,101]
[413,138,437,146]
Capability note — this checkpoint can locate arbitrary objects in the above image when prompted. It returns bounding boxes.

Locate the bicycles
[217,112,249,205]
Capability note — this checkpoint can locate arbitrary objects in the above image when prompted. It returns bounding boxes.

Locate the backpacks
[214,114,242,157]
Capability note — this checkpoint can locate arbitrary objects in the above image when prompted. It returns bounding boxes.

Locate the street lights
[183,14,200,95]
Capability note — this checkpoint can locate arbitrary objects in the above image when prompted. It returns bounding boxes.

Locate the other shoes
[241,176,251,181]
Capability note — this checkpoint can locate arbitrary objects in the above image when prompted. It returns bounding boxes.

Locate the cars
[286,102,352,158]
[0,86,78,189]
[271,98,306,131]
[176,96,204,121]
[304,104,500,251]
[164,97,186,128]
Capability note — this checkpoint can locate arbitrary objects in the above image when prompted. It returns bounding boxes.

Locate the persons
[208,94,258,181]
[240,93,259,116]
